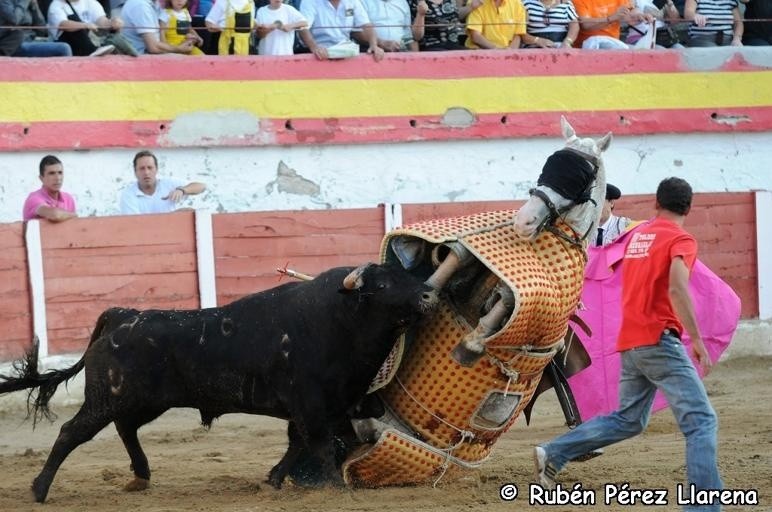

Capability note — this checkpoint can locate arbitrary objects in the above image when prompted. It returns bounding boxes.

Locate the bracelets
[175,186,186,196]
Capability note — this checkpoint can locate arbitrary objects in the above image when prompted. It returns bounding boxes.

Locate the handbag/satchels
[657,22,693,47]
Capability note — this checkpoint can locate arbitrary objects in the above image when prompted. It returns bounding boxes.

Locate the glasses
[544,7,550,25]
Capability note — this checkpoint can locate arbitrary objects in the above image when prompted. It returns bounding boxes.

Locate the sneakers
[533,446,557,492]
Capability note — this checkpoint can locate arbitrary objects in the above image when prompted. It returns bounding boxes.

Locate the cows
[0,260,440,504]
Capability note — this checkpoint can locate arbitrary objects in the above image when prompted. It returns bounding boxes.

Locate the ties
[596,228,603,246]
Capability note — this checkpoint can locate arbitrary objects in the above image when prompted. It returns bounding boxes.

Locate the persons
[587,184,634,248]
[23,156,77,223]
[0,0,771,62]
[119,150,206,218]
[533,176,723,511]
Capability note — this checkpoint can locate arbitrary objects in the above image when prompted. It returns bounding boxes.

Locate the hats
[606,184,621,200]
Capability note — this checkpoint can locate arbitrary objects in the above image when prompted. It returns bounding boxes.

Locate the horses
[338,114,613,492]
